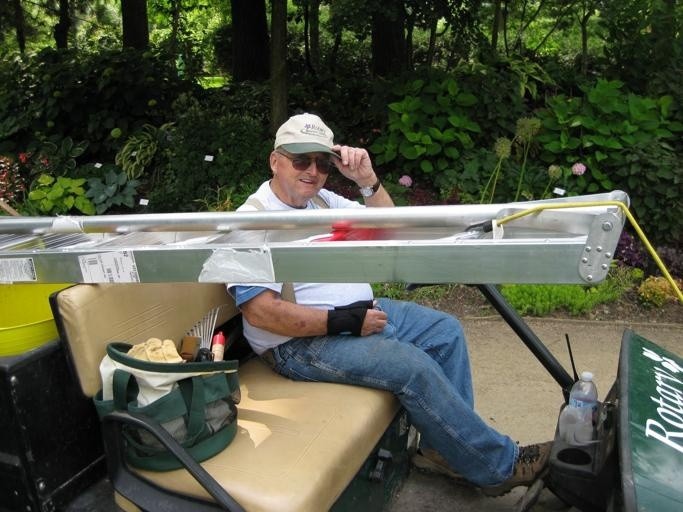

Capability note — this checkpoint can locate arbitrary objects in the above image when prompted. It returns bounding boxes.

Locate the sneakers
[411,447,462,477]
[481,441,553,498]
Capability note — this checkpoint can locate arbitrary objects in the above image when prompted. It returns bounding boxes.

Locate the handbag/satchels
[94,342,240,472]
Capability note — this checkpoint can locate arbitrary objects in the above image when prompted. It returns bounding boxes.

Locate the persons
[223,111,554,498]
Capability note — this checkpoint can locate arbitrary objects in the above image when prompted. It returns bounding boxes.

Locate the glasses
[277,152,333,174]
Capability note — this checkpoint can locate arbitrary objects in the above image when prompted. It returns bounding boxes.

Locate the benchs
[48,281,421,512]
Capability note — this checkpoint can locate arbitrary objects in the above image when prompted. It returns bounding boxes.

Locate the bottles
[210,331,226,362]
[567,371,598,416]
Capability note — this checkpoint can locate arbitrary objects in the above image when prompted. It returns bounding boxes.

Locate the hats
[274,113,342,160]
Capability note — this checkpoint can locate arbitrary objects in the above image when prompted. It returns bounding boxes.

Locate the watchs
[359,178,380,198]
